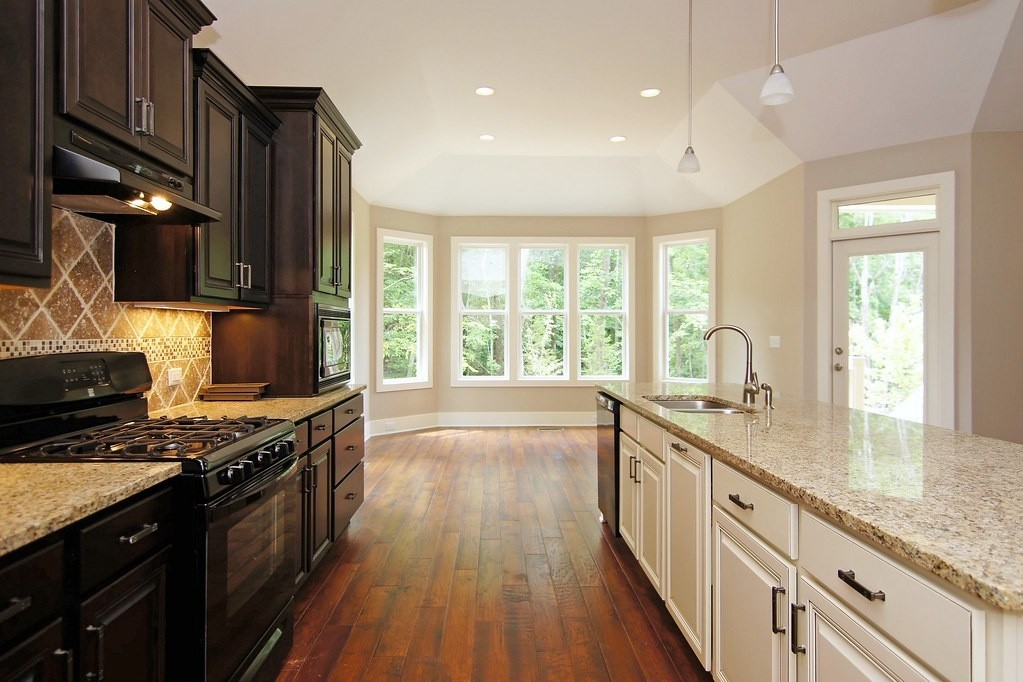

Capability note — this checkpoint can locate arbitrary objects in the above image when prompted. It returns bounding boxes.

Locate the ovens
[178,430,300,682]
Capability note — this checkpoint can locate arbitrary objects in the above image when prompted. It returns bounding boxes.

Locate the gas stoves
[1,352,294,472]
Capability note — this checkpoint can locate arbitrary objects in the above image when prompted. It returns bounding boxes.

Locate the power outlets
[768,335,781,349]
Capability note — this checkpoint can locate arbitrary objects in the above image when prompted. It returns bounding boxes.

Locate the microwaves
[211,303,352,398]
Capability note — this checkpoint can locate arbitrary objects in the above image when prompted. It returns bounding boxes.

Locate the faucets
[703,324,760,404]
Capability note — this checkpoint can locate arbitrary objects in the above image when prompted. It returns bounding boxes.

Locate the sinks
[644,392,767,418]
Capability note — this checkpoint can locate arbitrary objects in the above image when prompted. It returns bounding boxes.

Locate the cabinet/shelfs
[615,403,667,609]
[710,460,1022,682]
[664,430,712,682]
[0,2,367,682]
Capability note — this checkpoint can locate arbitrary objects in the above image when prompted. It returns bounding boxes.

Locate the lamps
[759,0,796,109]
[670,0,703,176]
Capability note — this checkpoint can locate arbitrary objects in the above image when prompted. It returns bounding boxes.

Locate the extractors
[49,145,224,225]
